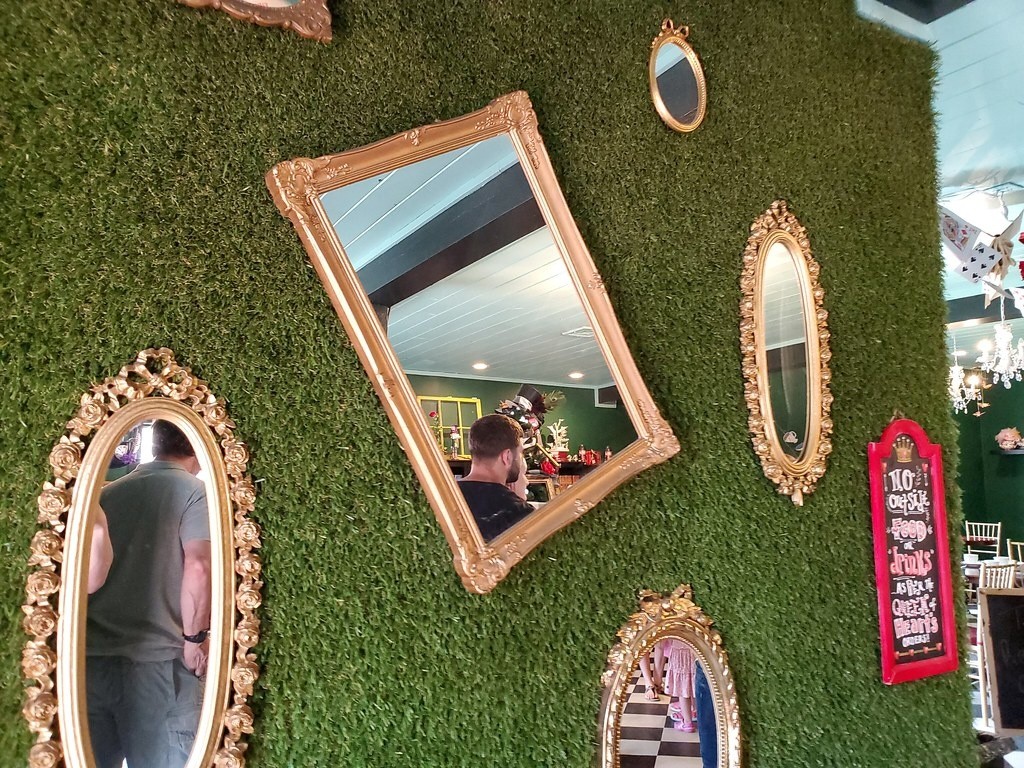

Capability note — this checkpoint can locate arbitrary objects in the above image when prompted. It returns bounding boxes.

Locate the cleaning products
[604,445,613,463]
[579,444,586,462]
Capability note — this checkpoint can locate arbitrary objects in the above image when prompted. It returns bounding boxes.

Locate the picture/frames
[867,418,961,686]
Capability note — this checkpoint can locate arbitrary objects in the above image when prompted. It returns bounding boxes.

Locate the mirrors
[595,584,743,768]
[649,18,707,133]
[268,81,685,597]
[735,196,834,512]
[176,0,334,45]
[18,344,266,768]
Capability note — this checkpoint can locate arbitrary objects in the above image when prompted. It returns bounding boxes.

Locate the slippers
[646,685,661,701]
[655,681,665,694]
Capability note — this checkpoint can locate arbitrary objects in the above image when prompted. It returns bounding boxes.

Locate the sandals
[668,701,694,711]
[670,711,697,722]
[676,720,696,733]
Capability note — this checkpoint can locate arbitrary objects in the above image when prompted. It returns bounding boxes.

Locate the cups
[964,554,1010,575]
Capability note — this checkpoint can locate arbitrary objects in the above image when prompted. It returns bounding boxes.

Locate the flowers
[428,411,442,445]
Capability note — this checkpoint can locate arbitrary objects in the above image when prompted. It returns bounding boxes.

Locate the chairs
[1006,538,1024,563]
[965,520,1002,603]
[977,561,1017,589]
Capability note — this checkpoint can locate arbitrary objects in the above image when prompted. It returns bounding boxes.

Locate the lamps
[948,182,1024,417]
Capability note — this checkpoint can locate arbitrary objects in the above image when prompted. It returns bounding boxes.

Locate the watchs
[182,629,207,643]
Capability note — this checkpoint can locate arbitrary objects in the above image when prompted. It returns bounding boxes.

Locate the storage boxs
[555,475,580,495]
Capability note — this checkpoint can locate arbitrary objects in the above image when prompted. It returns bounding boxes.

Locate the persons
[86,419,211,768]
[695,660,717,768]
[88,505,114,595]
[639,638,697,732]
[456,414,537,544]
